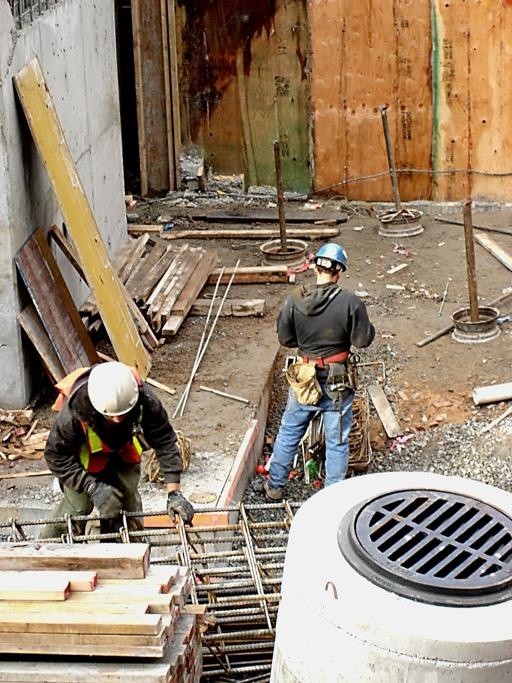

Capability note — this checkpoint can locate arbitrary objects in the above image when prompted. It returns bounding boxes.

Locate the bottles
[303,448,320,488]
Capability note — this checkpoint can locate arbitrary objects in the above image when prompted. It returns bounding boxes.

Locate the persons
[37,359,195,545]
[261,240,377,507]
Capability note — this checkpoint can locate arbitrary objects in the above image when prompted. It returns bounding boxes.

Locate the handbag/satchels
[284,361,323,408]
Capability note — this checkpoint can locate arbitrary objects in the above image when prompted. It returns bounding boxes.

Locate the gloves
[165,490,194,526]
[86,480,124,519]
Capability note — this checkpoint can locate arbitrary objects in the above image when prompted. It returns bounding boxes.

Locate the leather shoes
[263,480,283,503]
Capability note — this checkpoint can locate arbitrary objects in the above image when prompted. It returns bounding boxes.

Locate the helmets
[87,361,141,418]
[314,242,349,270]
